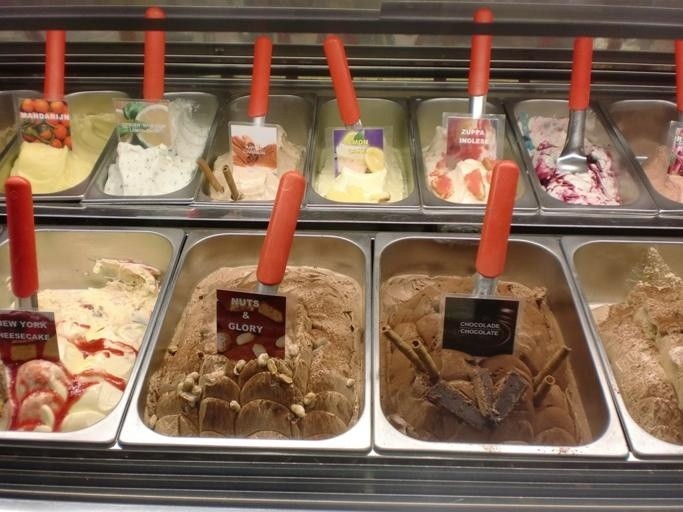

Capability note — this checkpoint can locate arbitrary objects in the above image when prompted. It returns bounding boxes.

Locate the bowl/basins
[302,95,423,207]
[497,92,658,212]
[0,86,43,159]
[0,86,138,202]
[192,92,317,205]
[408,95,540,211]
[371,230,630,458]
[116,229,374,452]
[79,89,223,201]
[594,93,683,212]
[558,235,683,458]
[0,227,188,445]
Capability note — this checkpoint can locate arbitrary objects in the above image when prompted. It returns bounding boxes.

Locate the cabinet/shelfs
[0,0,683,510]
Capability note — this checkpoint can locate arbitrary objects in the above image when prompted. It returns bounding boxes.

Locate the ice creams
[380,275,592,447]
[145,266,365,438]
[312,143,409,203]
[592,246,683,446]
[103,97,211,195]
[7,111,118,193]
[518,110,622,206]
[640,144,683,205]
[0,259,163,433]
[195,124,305,202]
[423,125,500,204]
